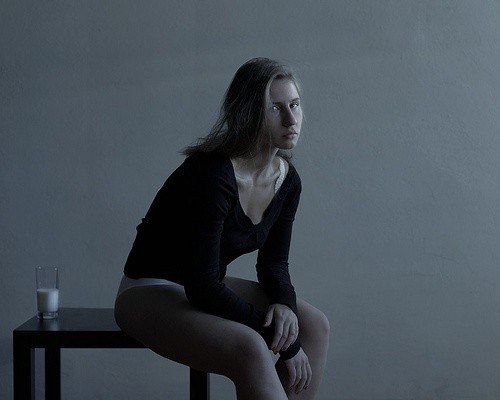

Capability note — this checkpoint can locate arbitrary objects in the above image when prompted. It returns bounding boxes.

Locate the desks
[14,308,210,400]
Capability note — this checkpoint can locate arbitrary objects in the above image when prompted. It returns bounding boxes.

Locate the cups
[35,265,59,319]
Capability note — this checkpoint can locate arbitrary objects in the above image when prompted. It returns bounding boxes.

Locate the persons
[114,57,330,400]
